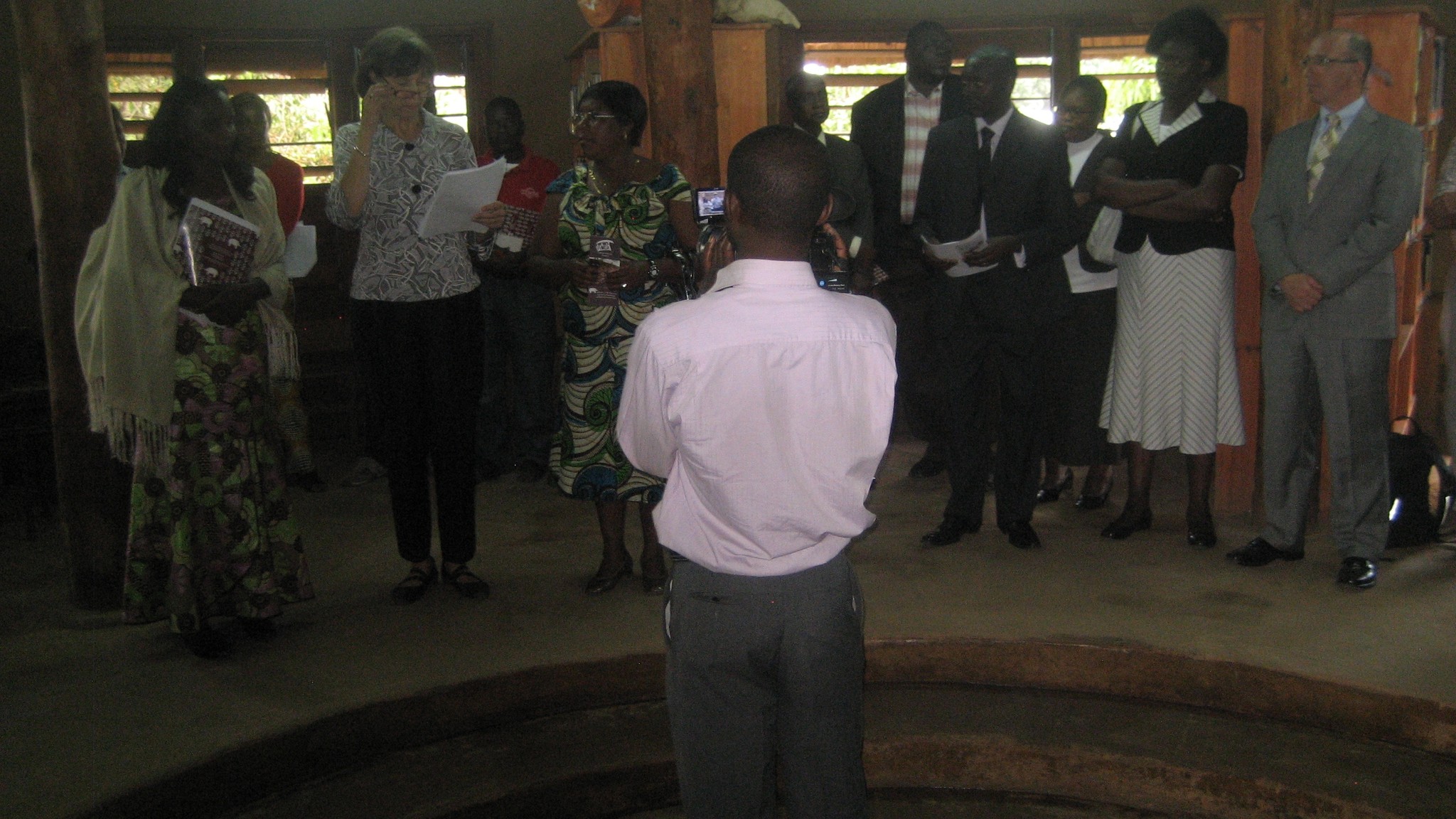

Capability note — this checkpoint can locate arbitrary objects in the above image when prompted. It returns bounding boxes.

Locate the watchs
[645,257,660,279]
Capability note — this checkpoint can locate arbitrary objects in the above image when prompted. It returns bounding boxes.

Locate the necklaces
[586,157,640,193]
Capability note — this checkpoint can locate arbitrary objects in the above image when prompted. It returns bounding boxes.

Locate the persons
[615,122,899,819]
[101,6,1454,660]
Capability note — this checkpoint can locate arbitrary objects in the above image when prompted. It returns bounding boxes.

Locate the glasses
[377,72,435,99]
[566,110,618,128]
[960,73,1007,89]
[911,38,954,53]
[1050,102,1100,118]
[1299,53,1360,71]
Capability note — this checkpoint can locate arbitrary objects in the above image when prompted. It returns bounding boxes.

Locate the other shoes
[985,473,1217,548]
[286,469,327,493]
[392,557,438,605]
[641,543,668,591]
[519,453,547,483]
[182,621,233,660]
[1385,514,1438,547]
[241,614,275,642]
[1436,494,1456,542]
[441,557,490,600]
[586,551,633,595]
[349,467,373,486]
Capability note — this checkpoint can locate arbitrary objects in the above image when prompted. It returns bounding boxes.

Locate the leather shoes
[909,453,946,477]
[999,520,1041,549]
[1226,536,1305,571]
[921,514,982,546]
[1335,556,1376,588]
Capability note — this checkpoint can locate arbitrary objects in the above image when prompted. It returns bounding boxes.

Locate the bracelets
[351,142,373,159]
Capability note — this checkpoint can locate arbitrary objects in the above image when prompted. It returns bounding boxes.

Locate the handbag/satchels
[1084,99,1155,265]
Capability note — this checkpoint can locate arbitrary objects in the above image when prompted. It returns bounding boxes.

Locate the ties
[1305,112,1341,205]
[968,125,996,237]
